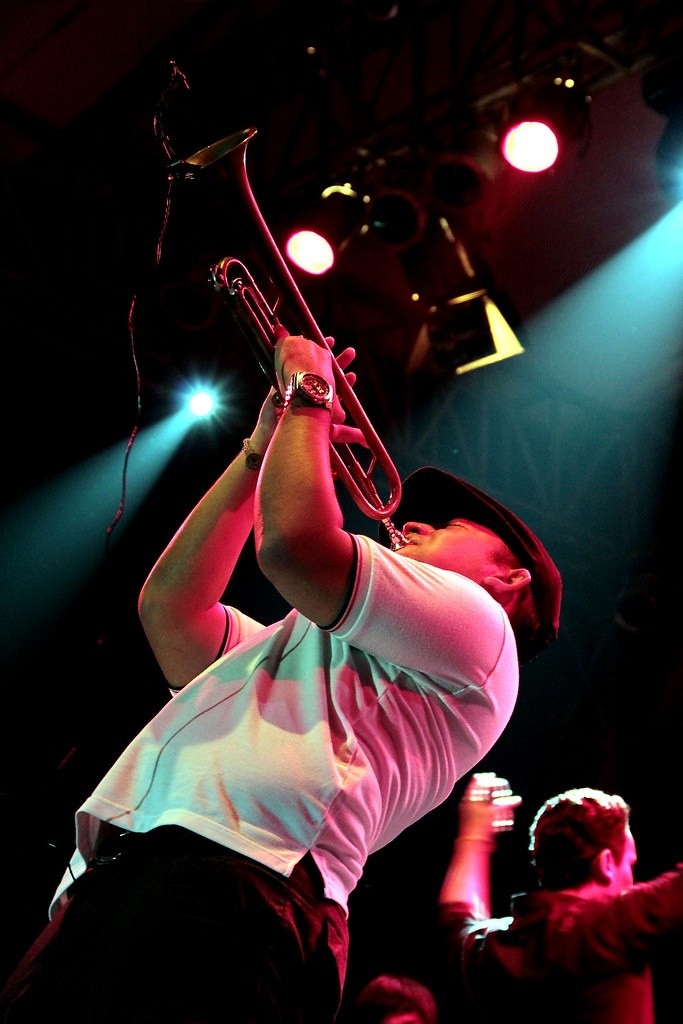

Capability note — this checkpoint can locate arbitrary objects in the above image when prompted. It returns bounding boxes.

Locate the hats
[378,465,561,663]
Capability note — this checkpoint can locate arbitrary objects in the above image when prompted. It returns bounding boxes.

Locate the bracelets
[243,437,261,470]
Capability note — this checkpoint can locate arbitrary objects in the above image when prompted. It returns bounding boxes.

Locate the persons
[439,771,683,1024]
[357,975,436,1024]
[0,335,560,1024]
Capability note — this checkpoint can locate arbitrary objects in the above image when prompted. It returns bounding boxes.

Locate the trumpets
[165,125,409,551]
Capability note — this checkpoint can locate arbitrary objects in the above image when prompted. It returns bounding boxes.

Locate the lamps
[286,33,626,377]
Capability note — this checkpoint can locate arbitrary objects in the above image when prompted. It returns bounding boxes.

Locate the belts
[90,828,142,866]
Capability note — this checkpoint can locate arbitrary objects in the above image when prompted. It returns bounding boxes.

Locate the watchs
[285,371,334,413]
